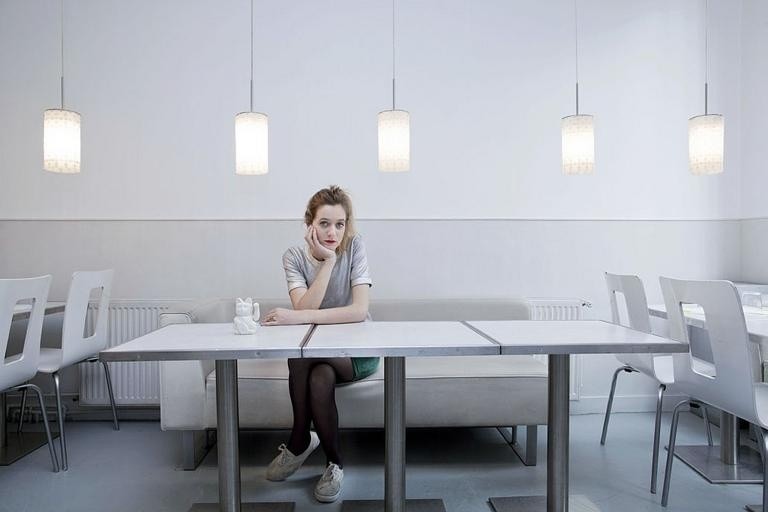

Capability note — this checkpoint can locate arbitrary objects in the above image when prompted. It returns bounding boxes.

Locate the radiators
[529,302,591,408]
[75,299,186,408]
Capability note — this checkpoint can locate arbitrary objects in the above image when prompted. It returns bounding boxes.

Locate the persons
[261,186,380,503]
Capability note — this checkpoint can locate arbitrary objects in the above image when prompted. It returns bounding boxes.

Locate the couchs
[161,301,550,472]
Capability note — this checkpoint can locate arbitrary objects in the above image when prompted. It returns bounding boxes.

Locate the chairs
[0,276,59,472]
[13,268,123,465]
[600,270,715,494]
[657,274,768,512]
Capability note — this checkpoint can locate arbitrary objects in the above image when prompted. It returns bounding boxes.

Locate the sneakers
[266,431,320,480]
[314,461,343,503]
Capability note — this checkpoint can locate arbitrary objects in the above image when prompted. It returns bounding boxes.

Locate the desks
[645,295,768,487]
[0,299,66,467]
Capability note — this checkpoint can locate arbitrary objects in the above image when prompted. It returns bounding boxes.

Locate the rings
[267,316,275,322]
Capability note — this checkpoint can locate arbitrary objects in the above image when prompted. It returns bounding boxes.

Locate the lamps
[376,1,412,173]
[230,1,271,177]
[685,2,726,175]
[41,0,82,177]
[561,0,596,176]
